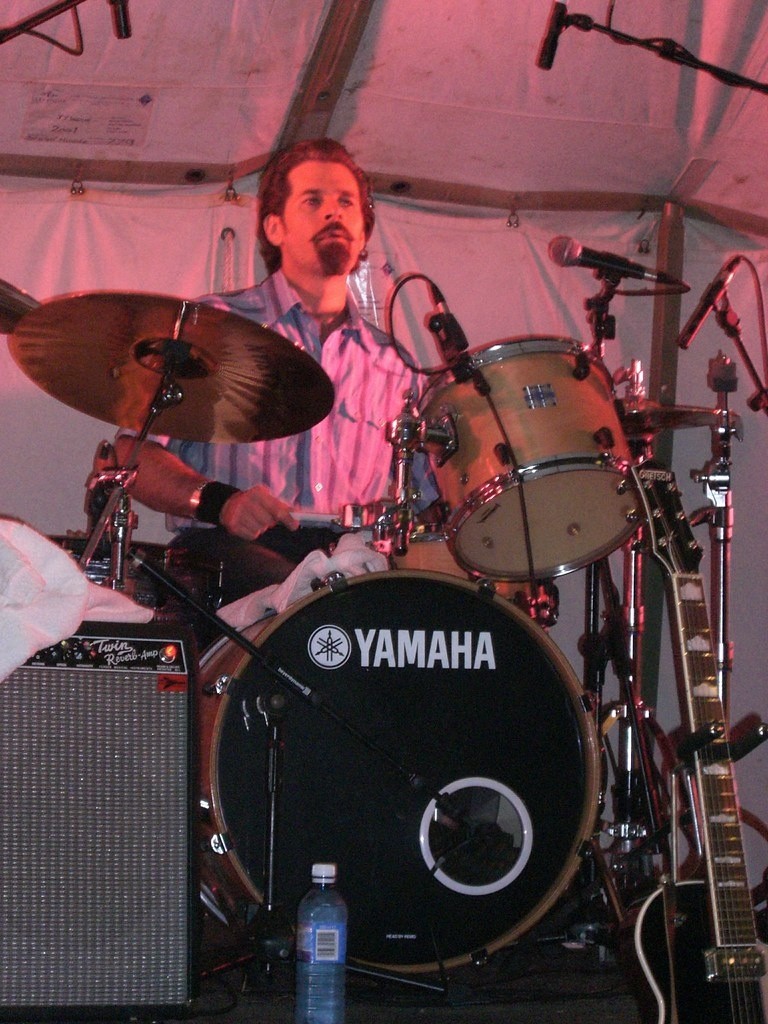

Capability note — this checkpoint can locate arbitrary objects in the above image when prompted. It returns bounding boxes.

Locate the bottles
[295,864,347,1024]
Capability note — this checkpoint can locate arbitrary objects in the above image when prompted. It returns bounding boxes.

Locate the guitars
[617,456,767,1024]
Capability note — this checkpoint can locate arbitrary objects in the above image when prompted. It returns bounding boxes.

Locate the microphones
[536,0,569,73]
[108,492,132,592]
[677,254,745,351]
[432,284,475,384]
[546,236,680,287]
[107,0,132,41]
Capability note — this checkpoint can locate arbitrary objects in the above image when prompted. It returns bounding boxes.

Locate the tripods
[475,291,746,977]
[127,548,515,1005]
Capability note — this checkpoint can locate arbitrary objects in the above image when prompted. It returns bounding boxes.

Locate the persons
[108,134,551,657]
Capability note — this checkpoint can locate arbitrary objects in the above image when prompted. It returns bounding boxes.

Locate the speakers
[0,615,198,1021]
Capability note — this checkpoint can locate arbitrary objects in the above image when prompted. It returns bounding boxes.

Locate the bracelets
[194,474,244,526]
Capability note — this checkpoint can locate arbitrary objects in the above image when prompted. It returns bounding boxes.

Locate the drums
[416,335,643,582]
[175,567,601,979]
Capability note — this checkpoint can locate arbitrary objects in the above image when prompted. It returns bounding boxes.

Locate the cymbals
[8,289,336,444]
[616,398,738,435]
